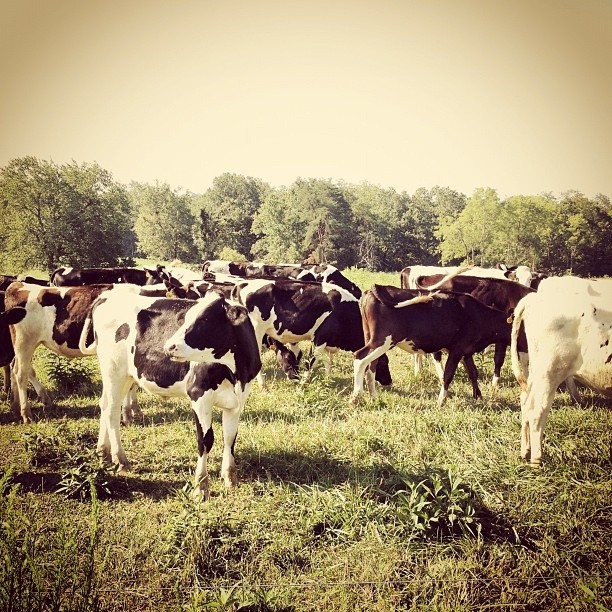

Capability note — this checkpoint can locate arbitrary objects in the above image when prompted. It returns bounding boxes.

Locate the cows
[243,263,302,278]
[352,285,528,408]
[201,260,248,279]
[278,264,361,302]
[78,292,261,501]
[51,265,167,288]
[0,273,48,291]
[537,277,612,404]
[413,275,538,387]
[500,265,545,292]
[511,293,612,464]
[231,280,391,393]
[0,290,26,393]
[186,280,301,381]
[401,264,539,307]
[6,282,170,424]
[161,268,199,297]
